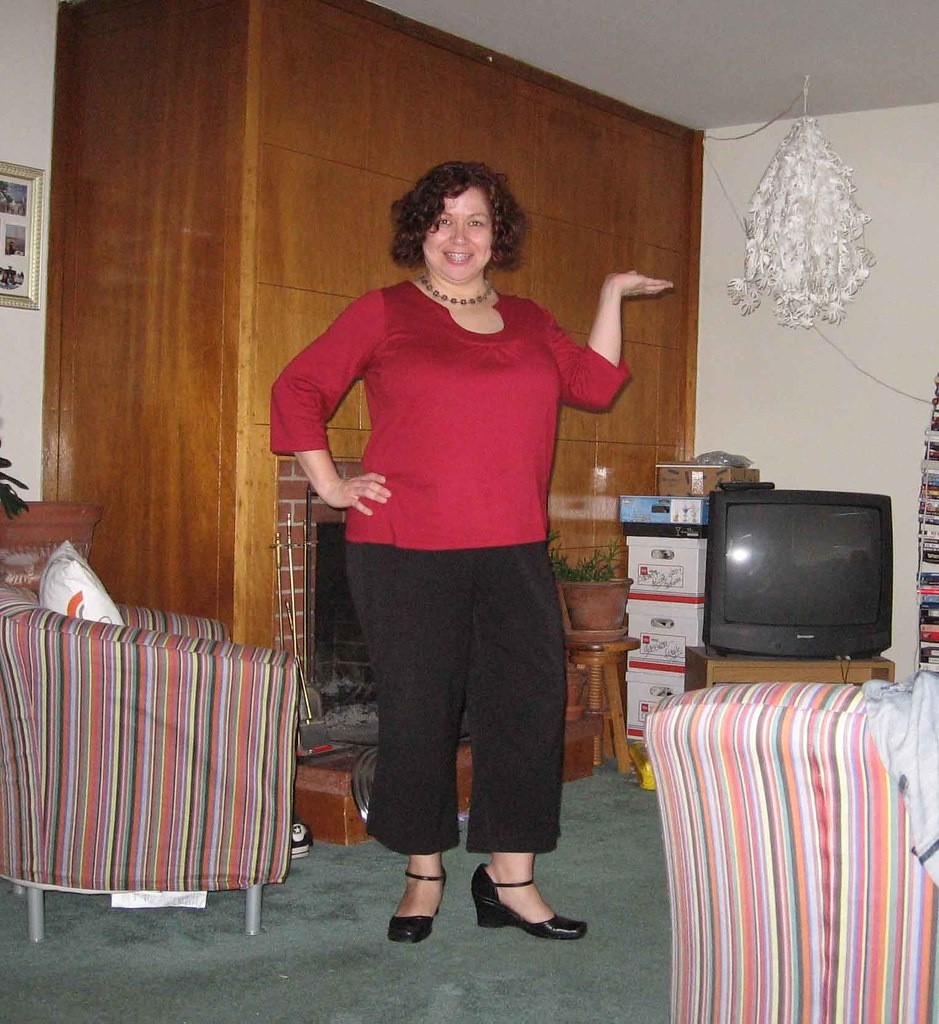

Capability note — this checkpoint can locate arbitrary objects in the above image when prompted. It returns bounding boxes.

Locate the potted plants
[546,533,634,630]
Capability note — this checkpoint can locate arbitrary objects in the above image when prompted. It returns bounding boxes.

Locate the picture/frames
[0,161,46,312]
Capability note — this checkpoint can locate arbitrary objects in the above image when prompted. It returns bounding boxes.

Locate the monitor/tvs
[707,492,892,663]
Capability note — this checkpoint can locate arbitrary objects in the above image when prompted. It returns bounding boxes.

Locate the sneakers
[289,823,313,859]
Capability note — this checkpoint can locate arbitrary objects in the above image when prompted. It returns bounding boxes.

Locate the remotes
[719,482,775,493]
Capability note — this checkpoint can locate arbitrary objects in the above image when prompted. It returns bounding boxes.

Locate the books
[916,374,939,676]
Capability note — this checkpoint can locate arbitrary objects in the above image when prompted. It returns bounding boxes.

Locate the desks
[685,644,895,692]
[564,638,641,772]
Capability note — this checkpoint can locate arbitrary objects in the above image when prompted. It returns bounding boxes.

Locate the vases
[0,500,102,591]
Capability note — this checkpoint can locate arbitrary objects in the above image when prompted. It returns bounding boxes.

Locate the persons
[271,162,672,943]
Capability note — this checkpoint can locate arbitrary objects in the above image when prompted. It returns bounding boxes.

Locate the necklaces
[420,274,492,304]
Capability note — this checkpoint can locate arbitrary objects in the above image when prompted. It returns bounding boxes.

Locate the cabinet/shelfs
[42,0,706,649]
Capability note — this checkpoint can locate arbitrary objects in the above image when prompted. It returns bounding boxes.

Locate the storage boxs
[618,467,759,740]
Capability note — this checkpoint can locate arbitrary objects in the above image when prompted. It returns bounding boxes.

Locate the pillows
[39,540,128,626]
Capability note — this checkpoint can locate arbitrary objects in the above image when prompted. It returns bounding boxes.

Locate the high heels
[470,862,588,940]
[387,865,447,942]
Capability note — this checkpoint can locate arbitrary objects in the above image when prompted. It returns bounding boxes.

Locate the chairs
[1,586,300,942]
[642,679,939,1023]
